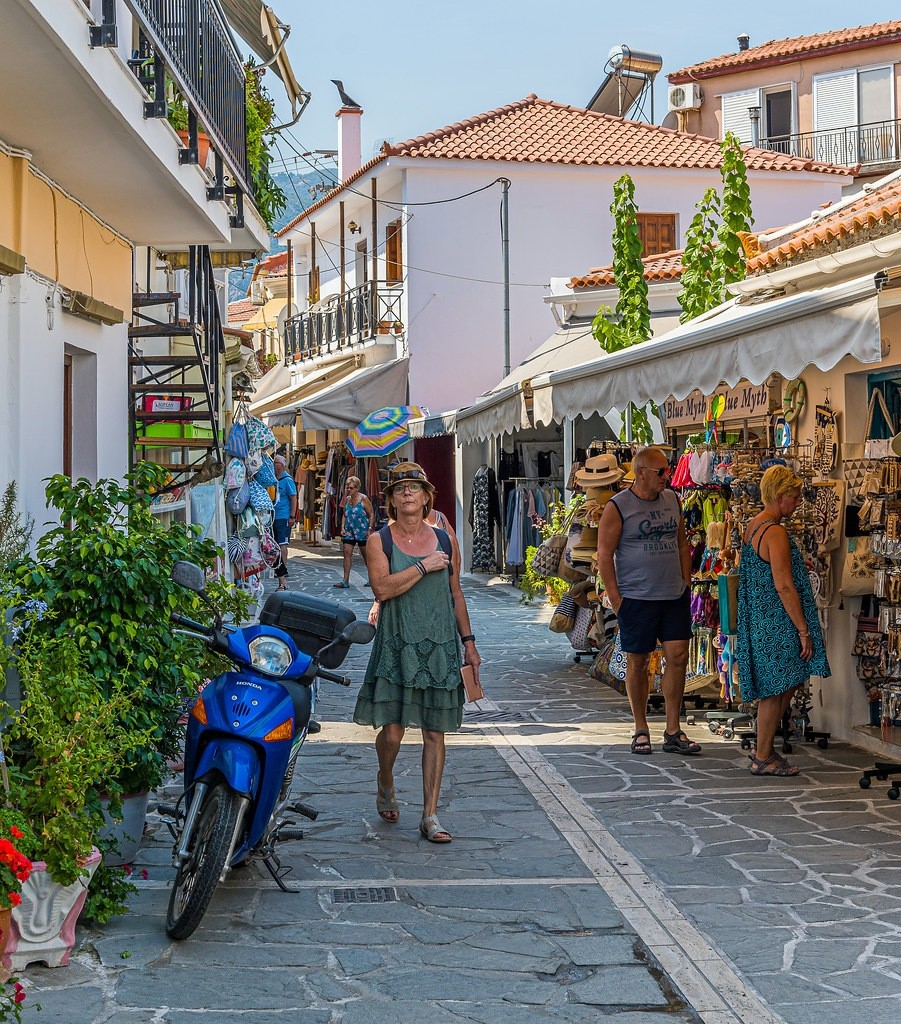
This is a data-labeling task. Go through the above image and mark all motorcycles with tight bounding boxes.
[157,559,379,943]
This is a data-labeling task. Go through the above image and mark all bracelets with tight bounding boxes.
[461,634,475,644]
[869,538,901,556]
[798,631,810,636]
[374,597,380,603]
[854,637,894,692]
[414,560,427,576]
[369,527,373,531]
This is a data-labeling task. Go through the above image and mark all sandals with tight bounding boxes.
[631,730,652,754]
[420,810,452,843]
[747,744,788,764]
[662,730,702,753]
[749,751,802,776]
[376,769,401,823]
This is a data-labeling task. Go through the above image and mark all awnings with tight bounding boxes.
[249,357,409,430]
[407,310,681,449]
[530,262,901,430]
[242,298,294,330]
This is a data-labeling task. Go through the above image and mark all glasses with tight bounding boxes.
[346,486,356,490]
[392,469,427,481]
[392,483,422,495]
[638,466,671,476]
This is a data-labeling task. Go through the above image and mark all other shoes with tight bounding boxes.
[274,573,289,577]
[364,582,371,587]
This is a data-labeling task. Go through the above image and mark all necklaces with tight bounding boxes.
[394,521,425,543]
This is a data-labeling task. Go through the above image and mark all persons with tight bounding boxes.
[264,456,297,592]
[736,464,832,777]
[353,462,481,842]
[597,448,702,754]
[333,476,375,588]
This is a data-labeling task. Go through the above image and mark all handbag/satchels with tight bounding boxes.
[659,633,719,693]
[841,387,901,680]
[530,501,582,577]
[549,580,627,696]
[717,572,739,636]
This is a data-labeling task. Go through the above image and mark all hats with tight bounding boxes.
[383,462,436,493]
[569,454,636,569]
[301,450,329,516]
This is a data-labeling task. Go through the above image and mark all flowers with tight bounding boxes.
[0,808,41,910]
[0,976,43,1024]
[0,588,163,924]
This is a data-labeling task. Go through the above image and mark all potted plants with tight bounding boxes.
[516,492,586,605]
[0,457,256,866]
[167,101,212,171]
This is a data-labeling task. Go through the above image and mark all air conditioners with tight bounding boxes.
[667,83,701,111]
[251,278,264,306]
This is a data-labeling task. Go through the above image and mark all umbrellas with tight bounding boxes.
[344,404,431,464]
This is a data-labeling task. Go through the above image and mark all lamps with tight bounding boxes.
[348,220,361,235]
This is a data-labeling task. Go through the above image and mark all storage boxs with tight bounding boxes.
[258,591,357,669]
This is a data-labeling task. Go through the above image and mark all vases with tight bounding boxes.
[2,843,103,971]
[0,906,11,985]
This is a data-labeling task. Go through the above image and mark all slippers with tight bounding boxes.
[275,583,288,592]
[333,581,350,588]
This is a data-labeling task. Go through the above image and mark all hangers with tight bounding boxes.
[542,445,551,452]
[528,444,540,452]
[504,441,513,448]
[515,478,564,493]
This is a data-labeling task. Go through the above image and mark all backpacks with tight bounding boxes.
[223,415,281,601]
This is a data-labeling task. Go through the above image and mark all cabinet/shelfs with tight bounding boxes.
[379,468,390,524]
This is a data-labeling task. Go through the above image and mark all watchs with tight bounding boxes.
[290,515,295,519]
